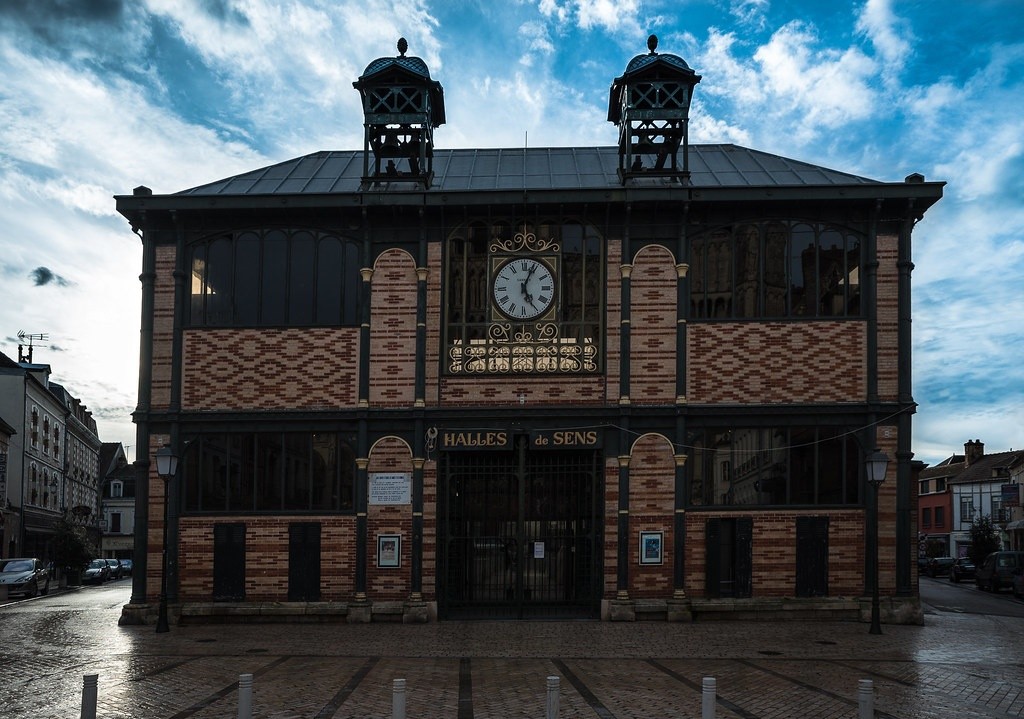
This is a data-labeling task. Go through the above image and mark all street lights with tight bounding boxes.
[865,449,893,633]
[153,443,180,632]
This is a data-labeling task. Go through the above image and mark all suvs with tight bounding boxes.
[0,558,49,599]
[106,559,124,580]
[119,559,134,577]
[82,561,106,585]
[94,559,112,581]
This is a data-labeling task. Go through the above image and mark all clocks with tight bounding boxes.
[492,259,558,321]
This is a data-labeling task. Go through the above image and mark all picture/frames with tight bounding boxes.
[638,529,665,567]
[376,533,401,569]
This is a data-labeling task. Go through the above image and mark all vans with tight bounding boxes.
[975,550,1024,593]
[926,557,957,578]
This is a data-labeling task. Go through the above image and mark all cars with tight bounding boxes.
[1011,566,1024,599]
[948,557,976,583]
